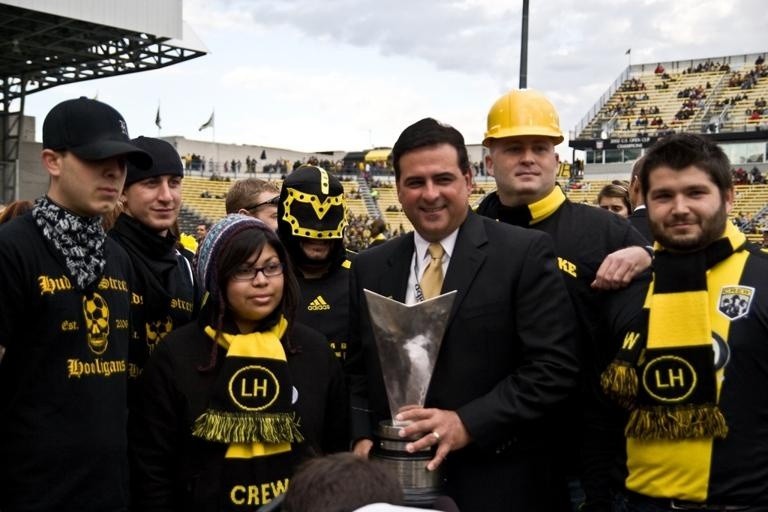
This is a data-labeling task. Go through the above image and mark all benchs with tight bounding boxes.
[578,64,768,138]
[557,177,768,258]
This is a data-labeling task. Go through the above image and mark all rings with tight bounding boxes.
[432,432,441,440]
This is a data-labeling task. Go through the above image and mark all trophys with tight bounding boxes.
[361,284,462,508]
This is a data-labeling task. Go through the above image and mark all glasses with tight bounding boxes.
[247,195,281,211]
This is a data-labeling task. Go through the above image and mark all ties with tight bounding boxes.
[420,242,445,300]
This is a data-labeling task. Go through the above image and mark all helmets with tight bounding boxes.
[482,90,565,148]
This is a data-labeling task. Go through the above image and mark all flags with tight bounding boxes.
[624,48,631,56]
[156,111,161,130]
[199,116,213,131]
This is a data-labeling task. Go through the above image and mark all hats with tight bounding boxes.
[194,212,279,292]
[42,96,153,172]
[125,136,184,189]
[278,165,348,241]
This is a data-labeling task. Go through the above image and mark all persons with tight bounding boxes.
[180,145,591,259]
[596,55,768,255]
[276,162,360,454]
[224,175,282,234]
[346,117,585,510]
[0,95,138,511]
[129,210,354,511]
[0,199,35,224]
[106,136,200,510]
[473,88,655,511]
[285,450,431,510]
[591,129,768,511]
